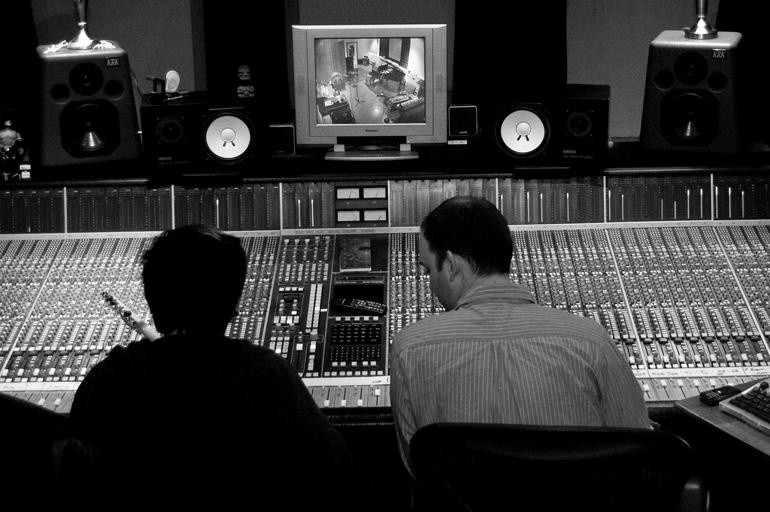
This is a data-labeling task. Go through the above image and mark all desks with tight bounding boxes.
[673,377,770,511]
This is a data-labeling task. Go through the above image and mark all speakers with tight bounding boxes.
[267,123,296,157]
[448,102,479,138]
[639,29,745,161]
[491,81,610,165]
[140,91,256,173]
[36,38,141,169]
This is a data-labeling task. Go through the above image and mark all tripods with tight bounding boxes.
[353,83,366,107]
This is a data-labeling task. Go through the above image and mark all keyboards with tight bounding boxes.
[718,377,770,434]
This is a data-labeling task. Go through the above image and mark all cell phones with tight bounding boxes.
[700,385,741,407]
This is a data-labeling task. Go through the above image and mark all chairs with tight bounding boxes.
[408,423,711,512]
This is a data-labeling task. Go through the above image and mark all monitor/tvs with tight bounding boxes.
[292,23,447,161]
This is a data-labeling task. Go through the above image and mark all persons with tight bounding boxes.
[385,194,659,483]
[70,222,352,512]
